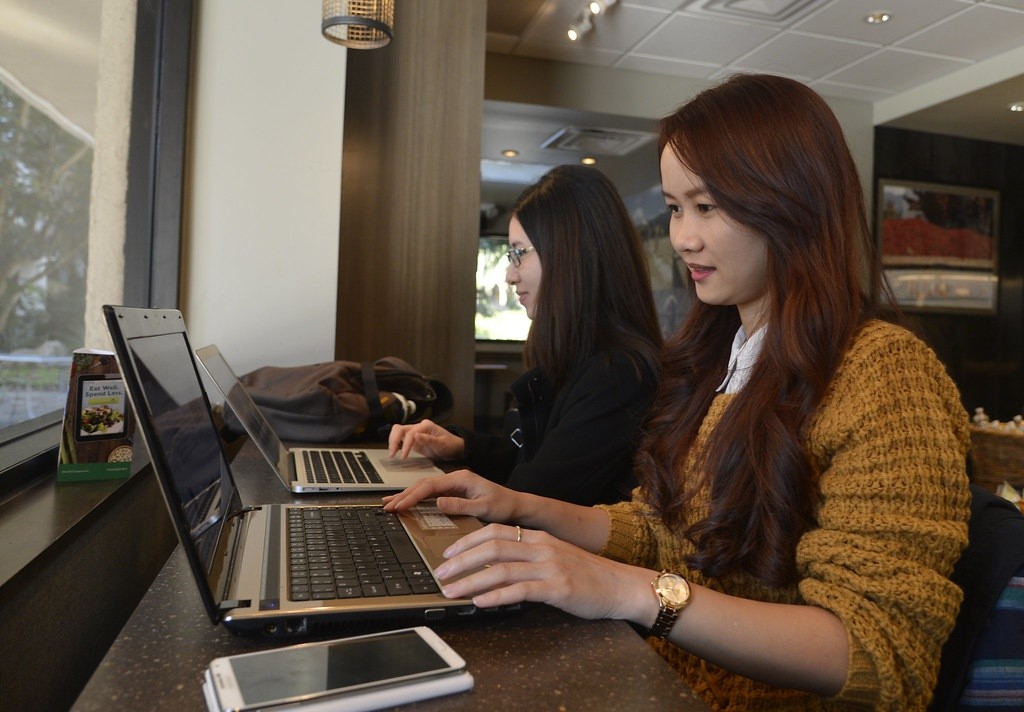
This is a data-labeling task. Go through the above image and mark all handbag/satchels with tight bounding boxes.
[221,357,455,446]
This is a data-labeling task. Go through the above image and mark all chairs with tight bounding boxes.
[927,482,1024,712]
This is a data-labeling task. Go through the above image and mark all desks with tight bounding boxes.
[67,439,713,712]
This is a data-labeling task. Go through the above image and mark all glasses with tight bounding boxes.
[507,245,536,268]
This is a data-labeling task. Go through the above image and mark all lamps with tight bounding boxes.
[590,0,617,16]
[567,7,596,41]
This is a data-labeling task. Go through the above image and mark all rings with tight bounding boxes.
[516,526,521,542]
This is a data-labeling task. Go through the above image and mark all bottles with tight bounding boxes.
[972,407,1024,433]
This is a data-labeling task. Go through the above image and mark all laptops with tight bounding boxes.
[194,344,448,493]
[102,304,523,639]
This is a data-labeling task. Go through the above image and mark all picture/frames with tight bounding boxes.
[875,177,1002,270]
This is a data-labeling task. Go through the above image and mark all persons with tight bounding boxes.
[380,72,972,712]
[386,165,664,506]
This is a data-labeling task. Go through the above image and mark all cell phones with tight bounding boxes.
[210,626,467,712]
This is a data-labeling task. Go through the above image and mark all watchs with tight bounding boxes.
[648,570,691,640]
